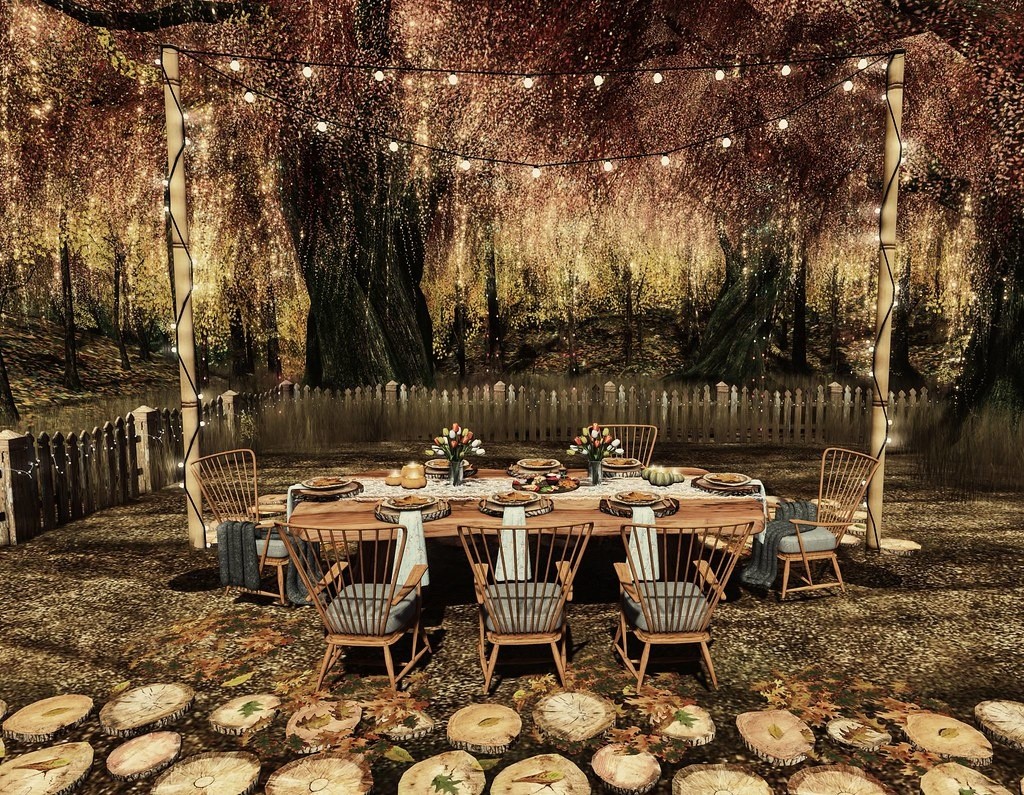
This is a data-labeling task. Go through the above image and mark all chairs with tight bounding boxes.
[457,521,594,694]
[273,520,430,699]
[612,521,755,694]
[741,448,881,599]
[587,425,658,468]
[188,449,331,605]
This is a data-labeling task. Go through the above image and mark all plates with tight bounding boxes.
[602,458,641,467]
[425,458,470,469]
[302,477,353,488]
[610,490,664,504]
[518,458,560,468]
[487,491,541,505]
[703,473,752,486]
[382,494,435,509]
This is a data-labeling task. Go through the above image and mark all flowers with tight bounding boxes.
[565,423,624,462]
[424,423,485,466]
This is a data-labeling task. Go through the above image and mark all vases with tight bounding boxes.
[449,462,464,486]
[588,461,602,485]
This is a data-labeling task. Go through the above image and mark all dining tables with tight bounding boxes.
[289,466,764,590]
